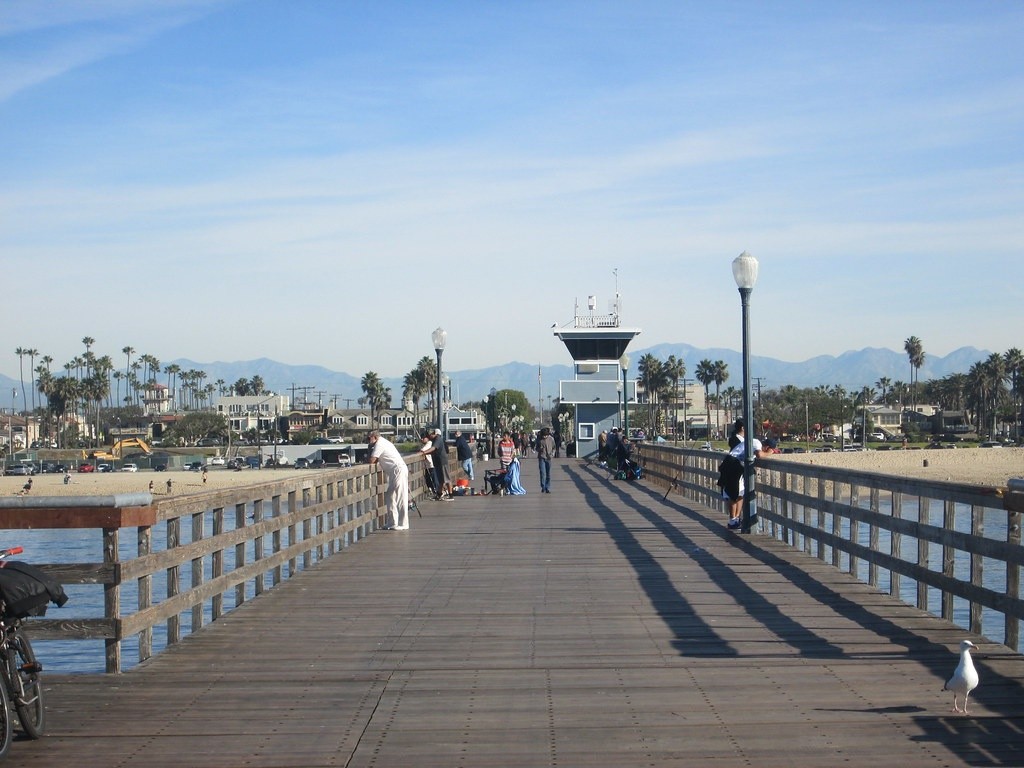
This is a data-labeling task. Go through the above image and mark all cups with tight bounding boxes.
[500,489,503,497]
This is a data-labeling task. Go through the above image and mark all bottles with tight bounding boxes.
[471,488,474,495]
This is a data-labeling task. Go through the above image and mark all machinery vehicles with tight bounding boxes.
[87,436,154,465]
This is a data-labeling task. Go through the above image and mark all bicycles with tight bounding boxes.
[0,544,46,768]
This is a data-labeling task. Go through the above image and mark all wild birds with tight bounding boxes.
[940,639,979,716]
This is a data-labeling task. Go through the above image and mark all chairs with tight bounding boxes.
[485,458,518,495]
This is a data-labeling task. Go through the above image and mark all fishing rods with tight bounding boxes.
[585,454,598,468]
[606,471,613,480]
[662,422,706,501]
[414,430,439,499]
[408,487,424,519]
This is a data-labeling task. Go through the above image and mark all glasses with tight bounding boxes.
[368,436,372,440]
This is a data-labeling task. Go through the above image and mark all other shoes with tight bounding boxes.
[542,487,546,493]
[467,476,474,480]
[728,520,743,529]
[393,526,409,530]
[444,498,455,502]
[440,491,446,498]
[381,524,394,530]
[546,488,550,493]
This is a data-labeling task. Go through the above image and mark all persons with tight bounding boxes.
[598,427,636,471]
[498,431,515,469]
[536,428,556,493]
[729,418,745,452]
[23,478,32,493]
[64,474,72,484]
[511,430,560,458]
[201,473,207,482]
[719,438,780,529]
[420,432,436,496]
[149,480,154,492]
[367,430,409,531]
[417,430,455,502]
[451,430,474,480]
[167,479,172,492]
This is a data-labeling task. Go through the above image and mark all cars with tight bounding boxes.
[309,435,344,445]
[182,461,208,472]
[5,462,70,476]
[196,433,288,447]
[77,463,94,473]
[121,463,138,473]
[337,453,351,467]
[777,431,886,454]
[154,463,167,471]
[228,453,326,470]
[922,430,1018,449]
[97,463,112,472]
[211,456,225,466]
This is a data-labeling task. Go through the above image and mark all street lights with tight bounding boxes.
[483,395,489,454]
[511,403,525,457]
[731,247,760,534]
[615,380,624,430]
[117,416,122,467]
[431,325,447,451]
[618,353,631,442]
[441,372,450,442]
[490,387,497,459]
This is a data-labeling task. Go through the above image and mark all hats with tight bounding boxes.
[435,428,441,435]
